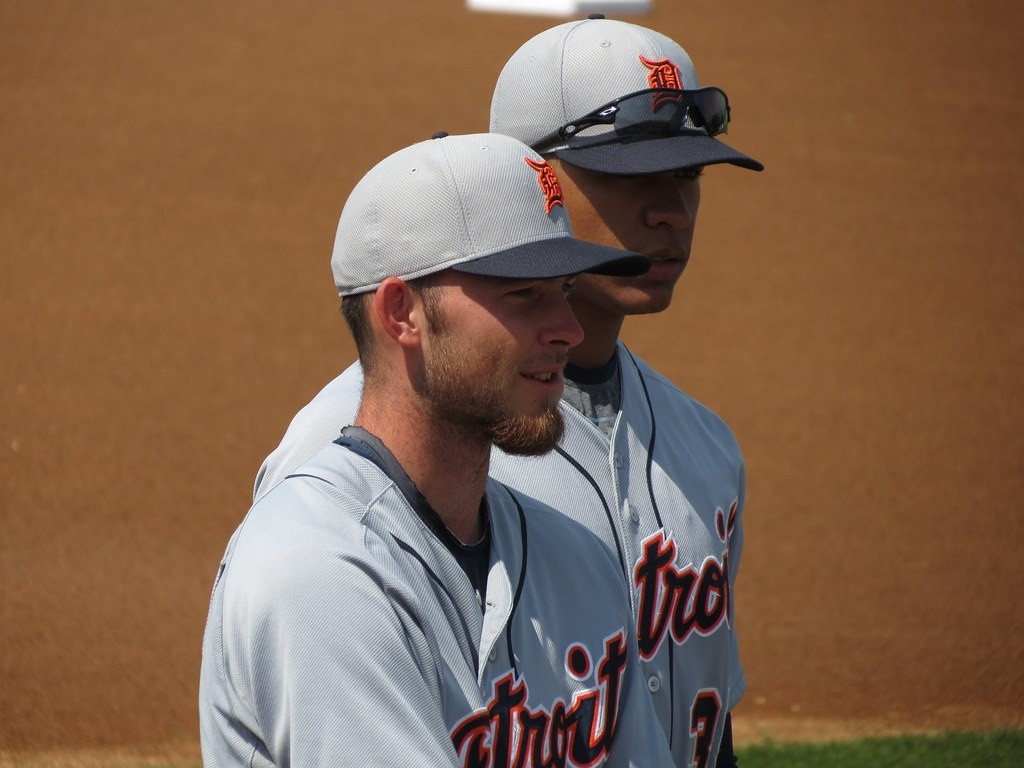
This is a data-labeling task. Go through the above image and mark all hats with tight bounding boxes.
[328,132,650,298]
[487,14,765,174]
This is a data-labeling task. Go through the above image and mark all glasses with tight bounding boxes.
[535,87,734,151]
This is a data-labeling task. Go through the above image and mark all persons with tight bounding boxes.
[197,133,676,768]
[247,15,764,768]
